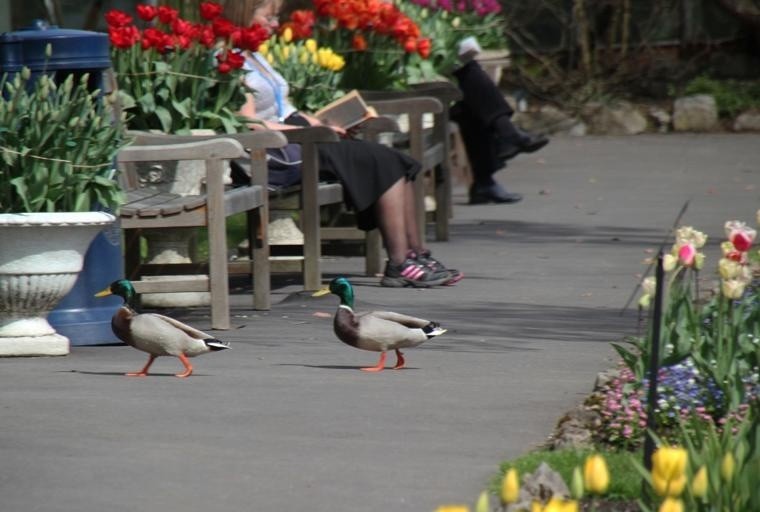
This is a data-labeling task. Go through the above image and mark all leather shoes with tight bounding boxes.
[499,132,548,159]
[470,184,520,203]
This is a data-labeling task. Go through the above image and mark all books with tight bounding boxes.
[459,37,482,64]
[314,89,378,136]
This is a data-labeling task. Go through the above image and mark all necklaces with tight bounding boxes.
[250,61,287,120]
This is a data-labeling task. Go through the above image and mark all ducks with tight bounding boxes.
[310,275,448,373]
[95,278,232,379]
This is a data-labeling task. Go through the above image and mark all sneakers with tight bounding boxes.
[381,256,452,287]
[407,248,463,284]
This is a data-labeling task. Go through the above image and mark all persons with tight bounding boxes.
[219,0,466,290]
[435,52,551,205]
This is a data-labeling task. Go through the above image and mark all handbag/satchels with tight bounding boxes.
[267,143,303,188]
